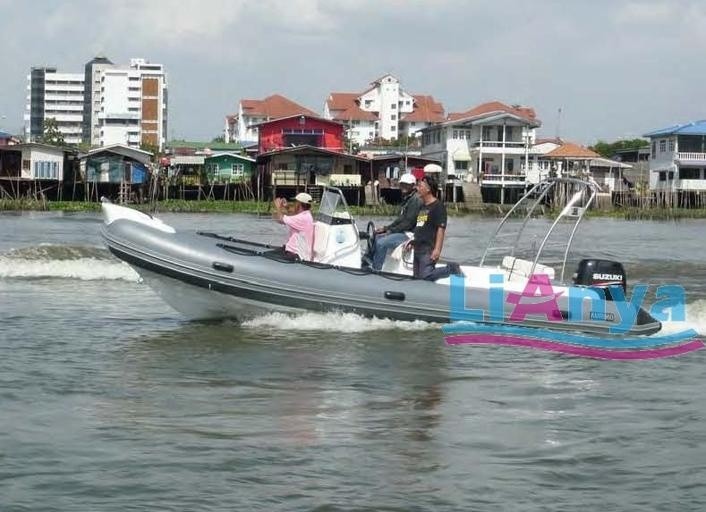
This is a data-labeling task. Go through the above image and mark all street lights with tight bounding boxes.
[0,116,7,119]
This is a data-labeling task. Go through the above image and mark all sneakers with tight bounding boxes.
[447,261,461,274]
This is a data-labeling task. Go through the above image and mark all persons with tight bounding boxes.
[361,172,422,276]
[401,175,462,282]
[269,192,315,261]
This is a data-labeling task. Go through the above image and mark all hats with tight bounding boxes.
[398,172,417,185]
[290,192,313,205]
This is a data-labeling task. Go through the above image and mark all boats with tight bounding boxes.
[92,176,669,339]
